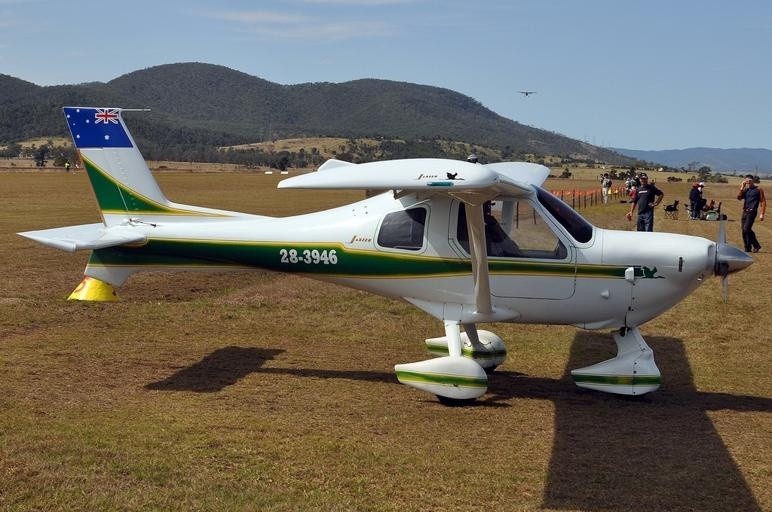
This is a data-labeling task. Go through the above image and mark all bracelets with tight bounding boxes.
[760,212,765,214]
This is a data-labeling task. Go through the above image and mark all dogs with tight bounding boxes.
[664,200,679,211]
[703,200,716,211]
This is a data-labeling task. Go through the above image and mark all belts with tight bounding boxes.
[744,209,754,211]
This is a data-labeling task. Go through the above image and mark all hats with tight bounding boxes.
[639,173,648,179]
[633,177,639,181]
[692,183,700,187]
[604,173,609,175]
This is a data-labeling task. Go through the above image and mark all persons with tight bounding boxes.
[751,175,761,188]
[736,174,767,254]
[688,181,727,221]
[624,173,664,231]
[598,165,656,206]
[64,159,83,176]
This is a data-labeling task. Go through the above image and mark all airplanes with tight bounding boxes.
[17,105,754,408]
[519,90,536,97]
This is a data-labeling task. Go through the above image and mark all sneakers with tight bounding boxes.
[745,245,761,253]
[690,217,696,220]
